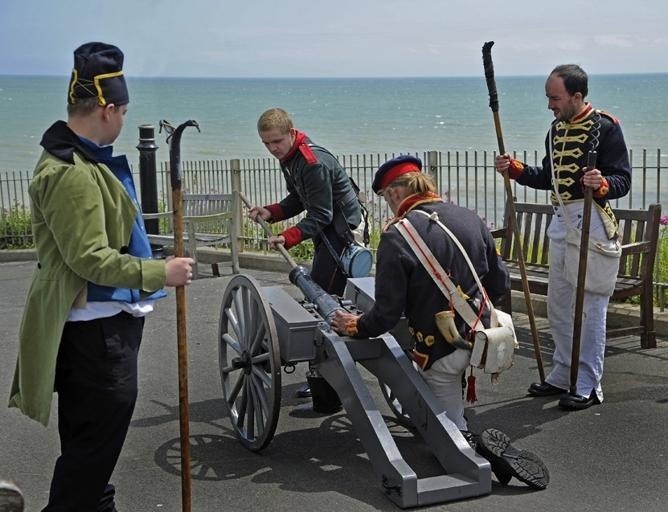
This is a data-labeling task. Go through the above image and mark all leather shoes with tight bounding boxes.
[476,429,550,491]
[558,391,596,412]
[295,384,312,398]
[526,381,568,396]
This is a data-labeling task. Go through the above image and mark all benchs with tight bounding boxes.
[489,195,662,355]
[141,188,241,281]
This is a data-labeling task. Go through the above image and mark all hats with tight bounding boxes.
[67,42,130,106]
[371,155,423,197]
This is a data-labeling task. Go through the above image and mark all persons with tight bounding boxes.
[495,64,632,410]
[248,107,362,397]
[6,41,195,512]
[329,155,550,492]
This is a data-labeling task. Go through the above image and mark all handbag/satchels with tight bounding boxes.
[469,326,514,375]
[350,208,372,251]
[490,308,519,351]
[562,227,623,298]
